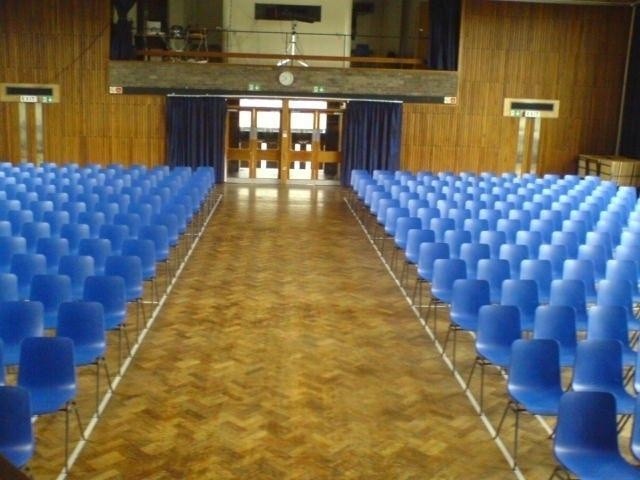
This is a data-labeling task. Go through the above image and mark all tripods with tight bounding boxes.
[278,33,309,67]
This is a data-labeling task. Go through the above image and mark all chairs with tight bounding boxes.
[182,23,209,64]
